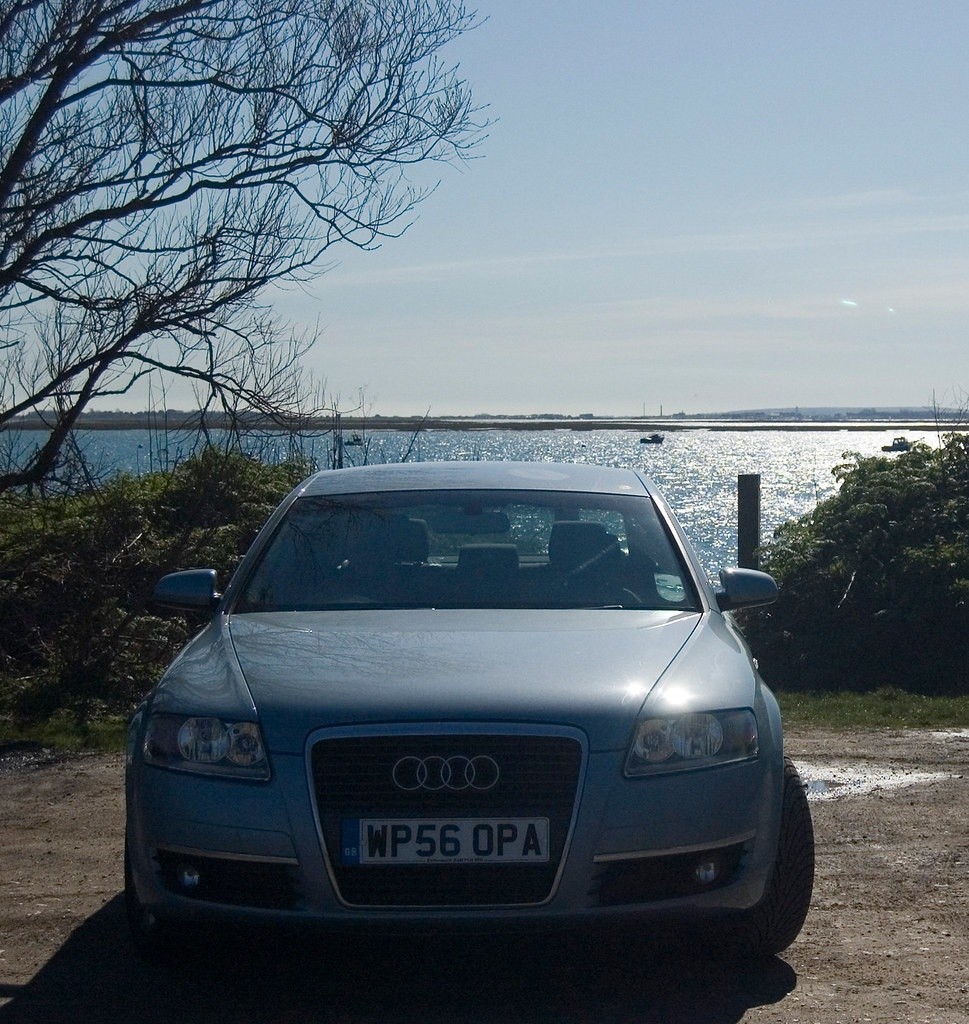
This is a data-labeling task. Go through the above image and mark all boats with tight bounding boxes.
[881,437,912,452]
[639,434,665,444]
[344,434,364,446]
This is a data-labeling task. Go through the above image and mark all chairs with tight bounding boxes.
[306,513,659,614]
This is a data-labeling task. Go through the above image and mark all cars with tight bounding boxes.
[122,460,816,972]
